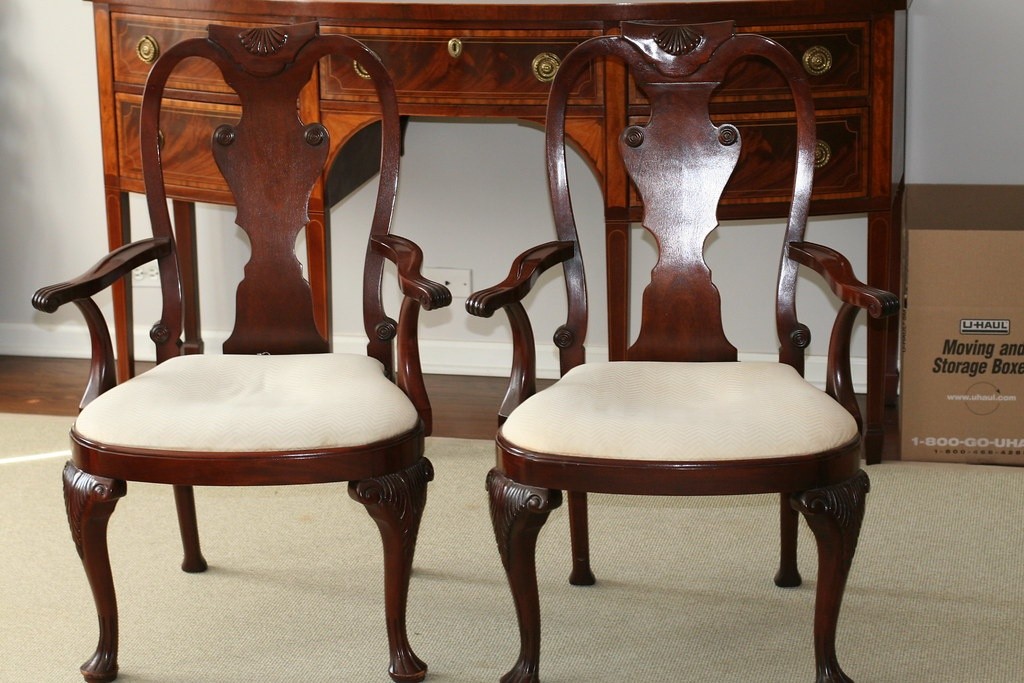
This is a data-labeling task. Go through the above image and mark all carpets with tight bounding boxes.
[0,409,1024,679]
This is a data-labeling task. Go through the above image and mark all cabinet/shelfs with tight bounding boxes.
[84,0,915,465]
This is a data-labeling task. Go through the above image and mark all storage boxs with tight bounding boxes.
[896,182,1024,467]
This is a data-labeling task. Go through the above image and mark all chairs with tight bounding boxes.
[30,22,450,681]
[464,24,896,683]
